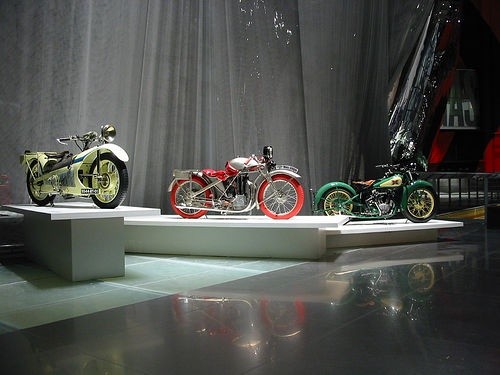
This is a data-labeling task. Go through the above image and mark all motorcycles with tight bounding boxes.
[330,258,455,323]
[18,117,130,208]
[169,282,308,352]
[309,163,440,225]
[167,142,305,220]
